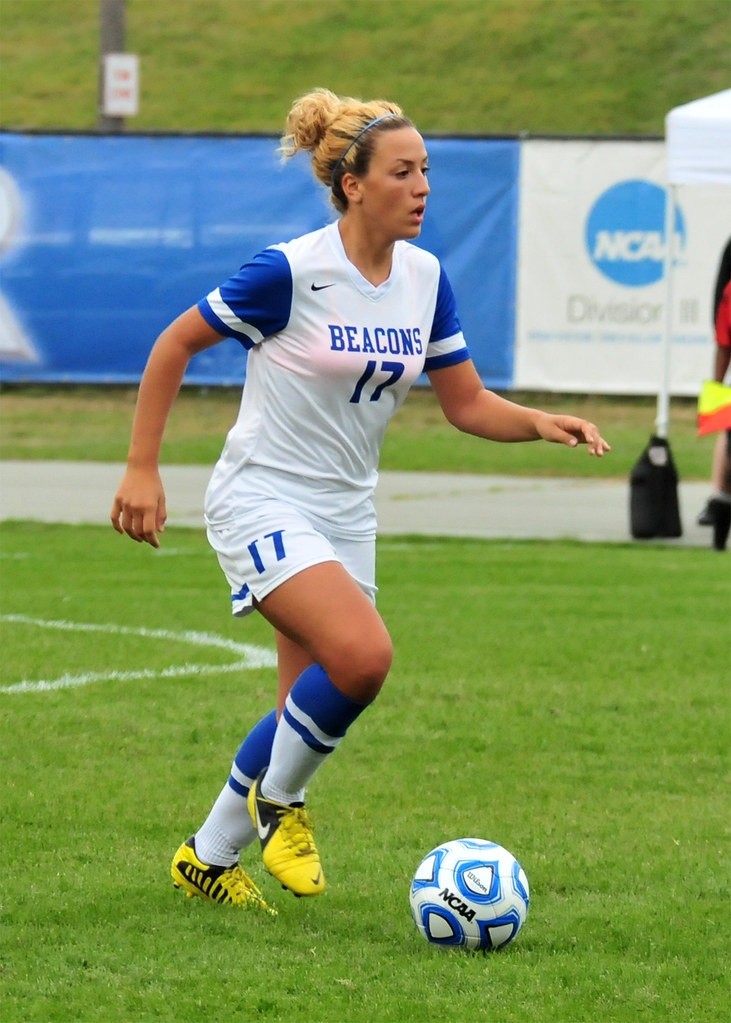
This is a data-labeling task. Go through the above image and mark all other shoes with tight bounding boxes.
[696,496,731,551]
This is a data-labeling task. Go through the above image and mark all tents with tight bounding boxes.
[654,88,731,437]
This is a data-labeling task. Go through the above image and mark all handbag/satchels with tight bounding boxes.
[630,436,683,539]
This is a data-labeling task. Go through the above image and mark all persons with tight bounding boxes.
[113,87,612,916]
[698,240,731,525]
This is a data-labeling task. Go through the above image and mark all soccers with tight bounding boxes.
[407,836,532,951]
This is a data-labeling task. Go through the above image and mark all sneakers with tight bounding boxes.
[169,833,278,921]
[245,766,326,898]
